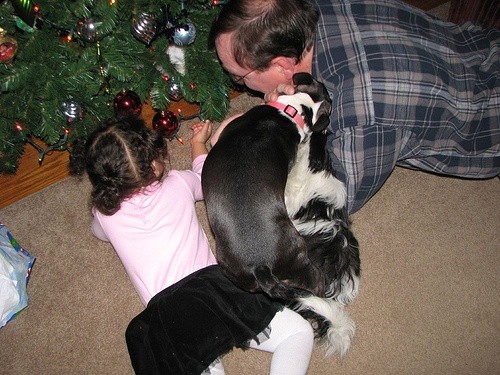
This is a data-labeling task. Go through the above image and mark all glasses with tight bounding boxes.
[229,60,267,86]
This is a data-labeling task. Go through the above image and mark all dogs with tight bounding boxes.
[200,72,361,359]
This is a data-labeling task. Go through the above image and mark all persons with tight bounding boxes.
[207,0,500,216]
[67,113,315,375]
